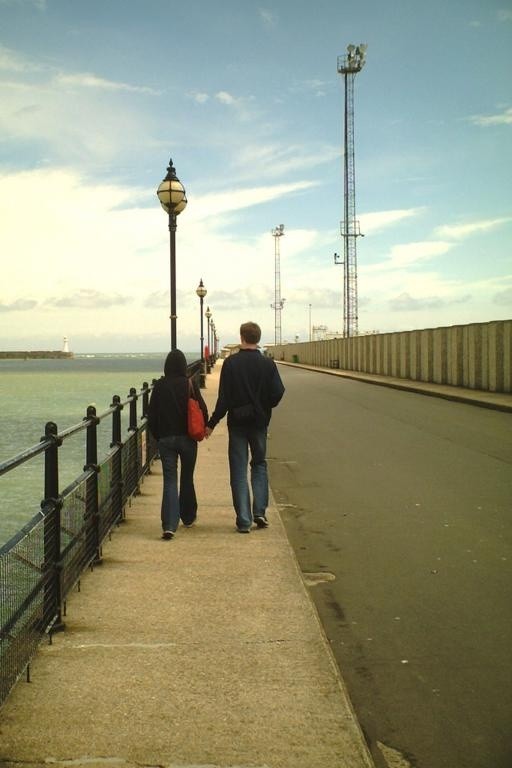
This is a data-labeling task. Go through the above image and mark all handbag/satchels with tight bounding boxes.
[187,398,205,441]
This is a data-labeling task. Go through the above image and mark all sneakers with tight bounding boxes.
[184,515,197,527]
[163,530,175,539]
[236,526,251,533]
[253,516,269,528]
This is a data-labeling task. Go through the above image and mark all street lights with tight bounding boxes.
[158,158,187,351]
[195,279,207,360]
[205,307,218,355]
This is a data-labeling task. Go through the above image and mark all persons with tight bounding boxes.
[205,322,285,532]
[149,350,210,539]
